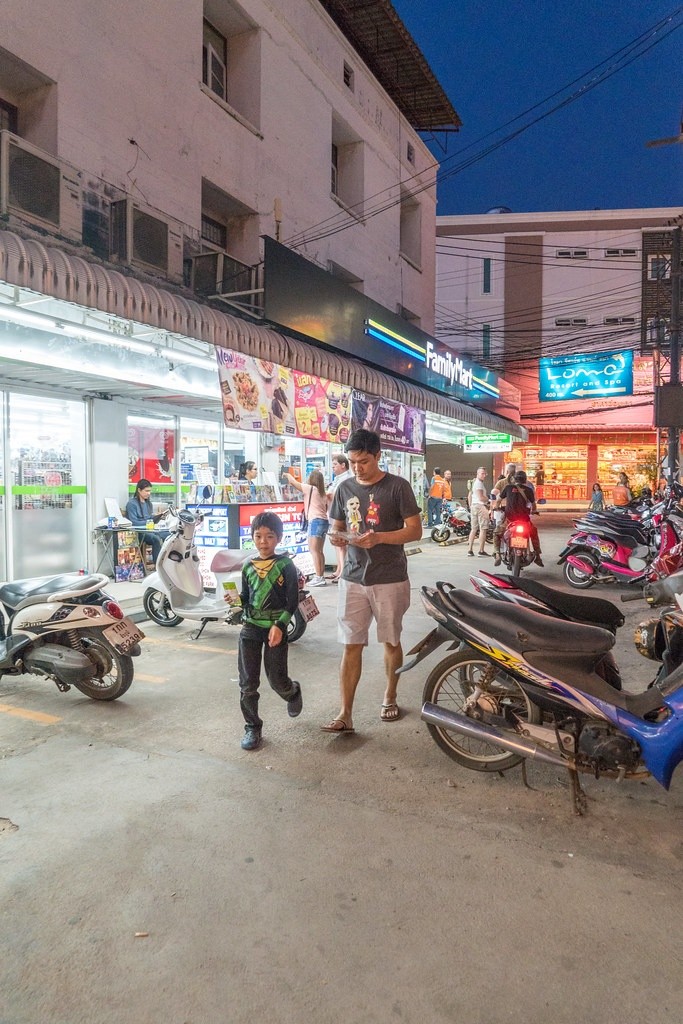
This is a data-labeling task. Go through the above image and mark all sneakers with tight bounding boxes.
[305,575,326,587]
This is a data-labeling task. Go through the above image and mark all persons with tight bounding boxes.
[612,472,634,506]
[641,484,651,497]
[321,428,422,733]
[324,453,353,583]
[466,467,491,557]
[223,512,303,749]
[423,467,453,529]
[492,463,544,568]
[588,483,607,511]
[282,470,329,586]
[535,466,546,484]
[126,479,171,561]
[238,461,258,485]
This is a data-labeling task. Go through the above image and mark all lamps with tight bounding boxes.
[0,303,219,369]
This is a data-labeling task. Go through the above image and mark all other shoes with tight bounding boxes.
[494,557,501,566]
[467,550,474,556]
[534,557,544,568]
[478,551,491,557]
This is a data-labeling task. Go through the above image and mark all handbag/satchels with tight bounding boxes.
[299,509,308,532]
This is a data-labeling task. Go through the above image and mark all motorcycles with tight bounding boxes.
[395,568,683,817]
[430,506,497,544]
[555,482,683,589]
[486,494,546,578]
[142,484,322,644]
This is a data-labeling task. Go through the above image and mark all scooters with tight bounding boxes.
[0,570,145,701]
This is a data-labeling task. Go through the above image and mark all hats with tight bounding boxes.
[516,471,527,478]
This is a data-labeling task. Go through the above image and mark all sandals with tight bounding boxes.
[287,682,302,718]
[332,576,340,582]
[241,727,263,750]
[324,575,336,579]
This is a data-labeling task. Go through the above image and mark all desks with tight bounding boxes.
[94,526,176,582]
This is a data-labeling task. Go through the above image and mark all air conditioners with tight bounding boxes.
[190,250,256,315]
[0,130,87,246]
[107,196,180,282]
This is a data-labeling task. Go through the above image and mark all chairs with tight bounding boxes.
[112,511,152,581]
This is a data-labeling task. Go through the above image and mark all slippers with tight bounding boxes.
[319,719,355,733]
[379,700,399,722]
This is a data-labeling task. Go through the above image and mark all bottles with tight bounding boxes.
[150,520,154,529]
[146,520,150,530]
[161,518,166,529]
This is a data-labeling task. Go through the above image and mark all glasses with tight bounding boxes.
[249,467,257,472]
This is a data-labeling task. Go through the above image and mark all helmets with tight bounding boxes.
[634,619,664,662]
